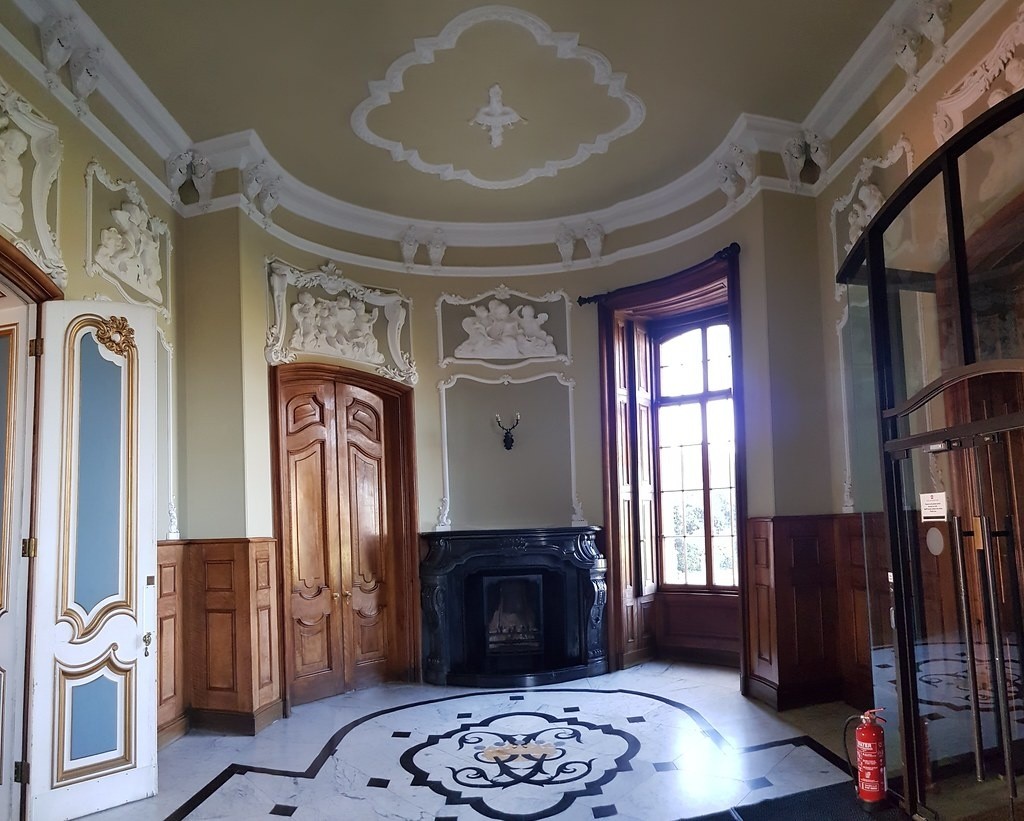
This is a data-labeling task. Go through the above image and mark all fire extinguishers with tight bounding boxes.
[840,705,893,802]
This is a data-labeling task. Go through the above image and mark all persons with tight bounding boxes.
[112,202,164,283]
[470,299,549,342]
[844,184,887,253]
[0,117,28,215]
[988,61,1023,109]
[292,291,378,364]
